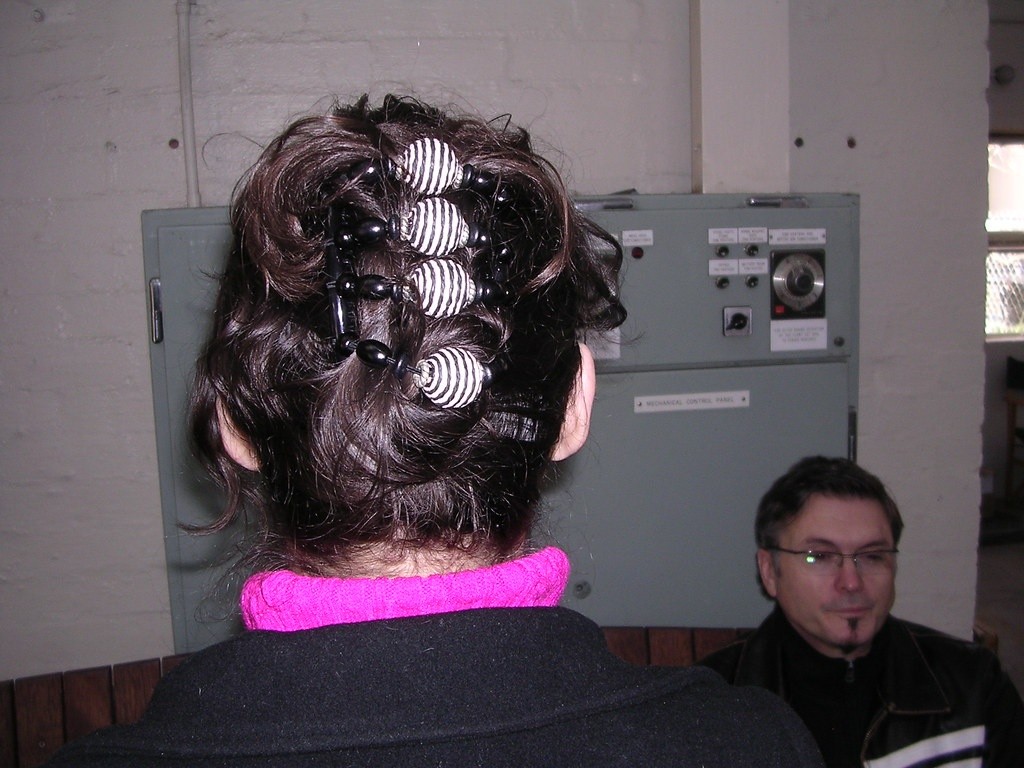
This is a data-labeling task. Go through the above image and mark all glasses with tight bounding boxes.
[771,548,899,577]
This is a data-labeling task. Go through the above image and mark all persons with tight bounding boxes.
[701,453,1024,768]
[0,92,823,767]
